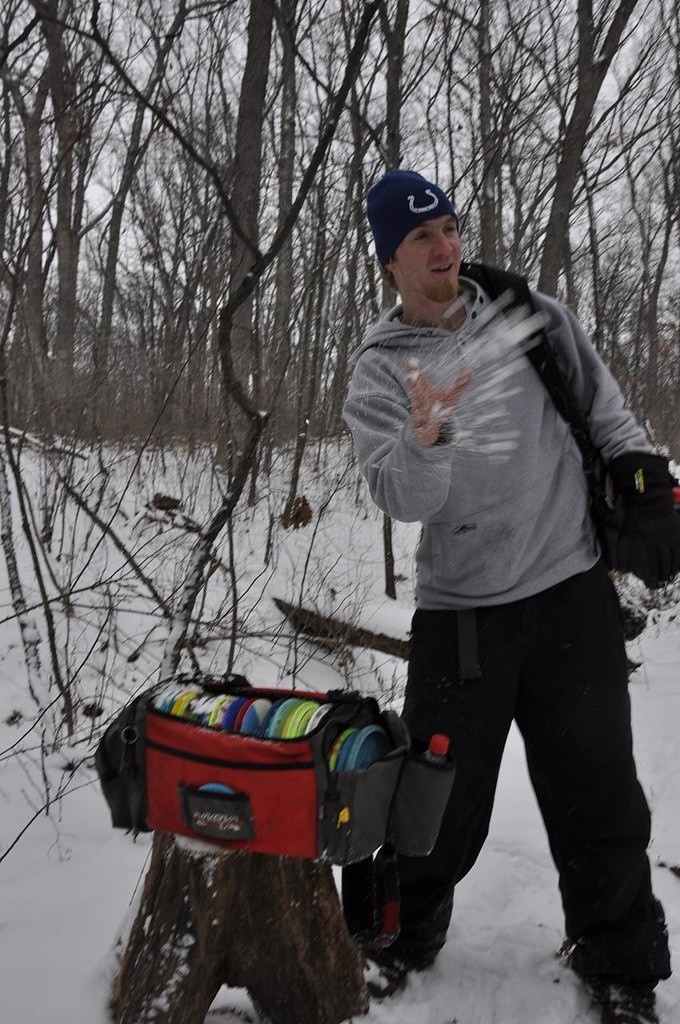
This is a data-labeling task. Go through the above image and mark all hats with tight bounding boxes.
[366,170,461,264]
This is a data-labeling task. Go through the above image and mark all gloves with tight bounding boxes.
[607,450,678,588]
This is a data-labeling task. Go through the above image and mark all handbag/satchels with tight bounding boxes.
[95,673,455,866]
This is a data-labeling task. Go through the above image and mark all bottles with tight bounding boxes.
[420,733,450,765]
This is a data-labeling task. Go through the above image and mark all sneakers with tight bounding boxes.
[596,985,656,1024]
[364,952,436,996]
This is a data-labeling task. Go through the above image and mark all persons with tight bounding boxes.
[338,164,674,1024]
